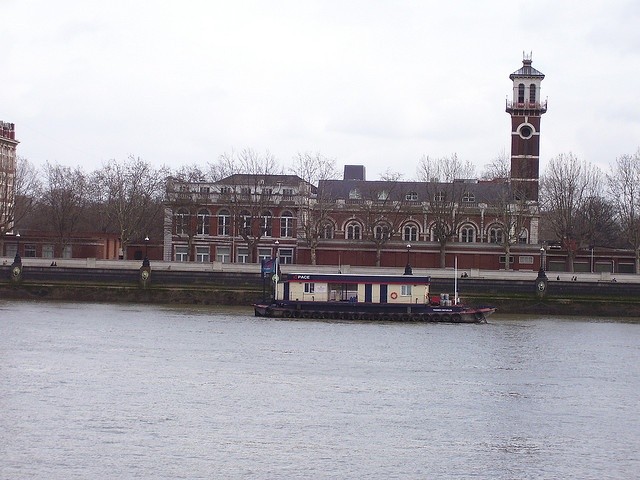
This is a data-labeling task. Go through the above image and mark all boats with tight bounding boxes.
[249,273,500,324]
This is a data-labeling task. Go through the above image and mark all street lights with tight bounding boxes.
[14,233,21,263]
[142,237,150,267]
[536,248,548,279]
[273,239,281,273]
[404,244,413,275]
